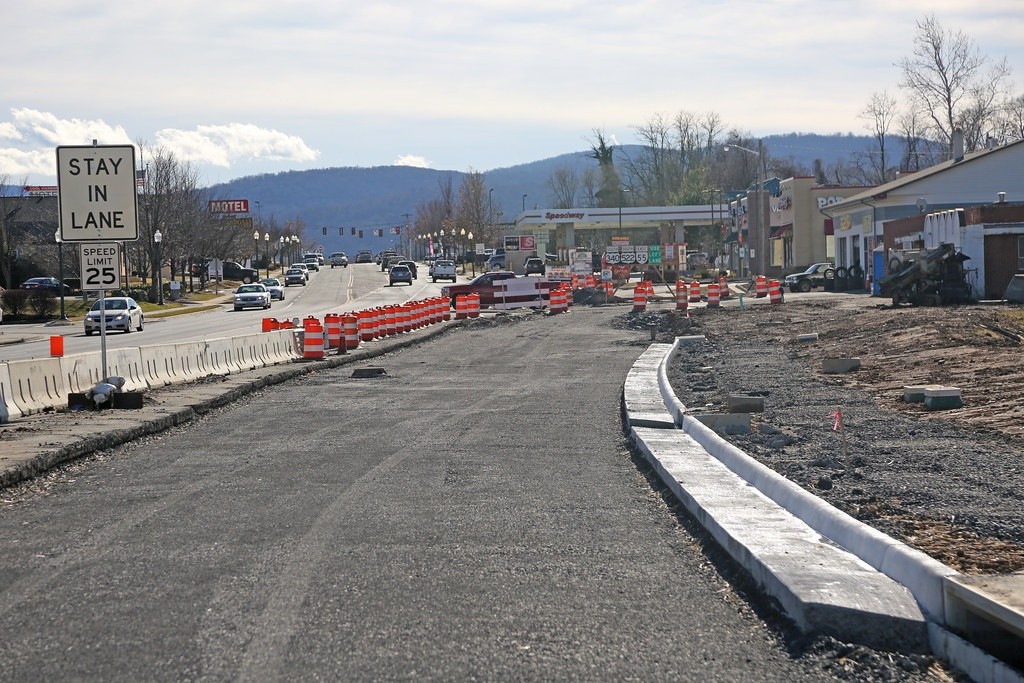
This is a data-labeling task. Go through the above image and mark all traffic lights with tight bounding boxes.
[396,227,400,235]
[379,230,383,237]
[359,230,363,238]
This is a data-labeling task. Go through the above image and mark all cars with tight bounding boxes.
[686,250,709,270]
[428,262,434,276]
[290,264,309,281]
[284,268,307,286]
[374,249,419,286]
[259,278,285,300]
[232,284,271,310]
[303,253,324,265]
[84,297,144,336]
[457,249,506,270]
[535,250,557,262]
[354,249,372,263]
[20,276,70,296]
[304,258,320,272]
[328,251,348,268]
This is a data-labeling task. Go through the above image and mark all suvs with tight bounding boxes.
[523,257,546,276]
[784,263,833,293]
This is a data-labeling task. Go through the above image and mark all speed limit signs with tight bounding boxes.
[79,242,121,290]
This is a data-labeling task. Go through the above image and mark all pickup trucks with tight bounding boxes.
[441,269,550,310]
[432,260,457,283]
[424,252,457,262]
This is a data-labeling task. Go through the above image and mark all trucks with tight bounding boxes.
[191,261,259,284]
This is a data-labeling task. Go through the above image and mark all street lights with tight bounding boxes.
[265,233,269,278]
[468,232,475,278]
[618,190,630,248]
[489,188,494,246]
[523,194,527,212]
[701,145,766,275]
[415,228,465,272]
[280,235,300,274]
[254,231,259,283]
